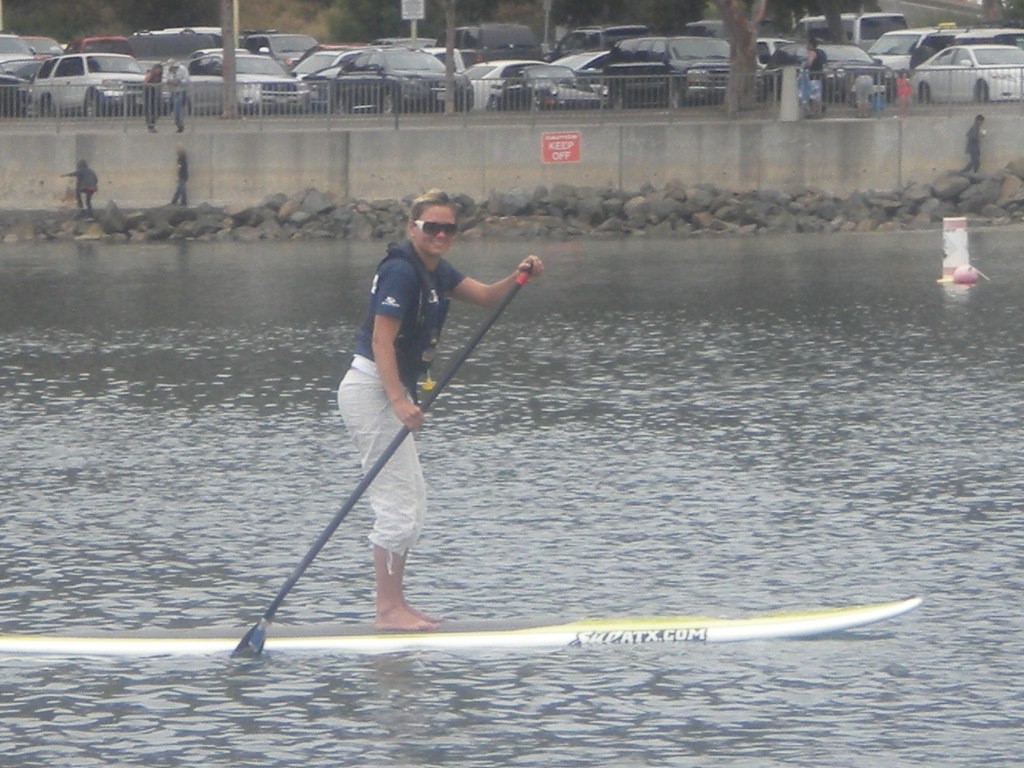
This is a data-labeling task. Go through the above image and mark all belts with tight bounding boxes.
[351,357,413,404]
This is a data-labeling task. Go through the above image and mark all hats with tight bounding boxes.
[167,59,179,70]
[774,41,787,49]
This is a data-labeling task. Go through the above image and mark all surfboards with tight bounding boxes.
[0,588,924,662]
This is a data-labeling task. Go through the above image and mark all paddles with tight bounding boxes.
[228,263,538,664]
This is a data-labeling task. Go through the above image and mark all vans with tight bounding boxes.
[789,12,909,52]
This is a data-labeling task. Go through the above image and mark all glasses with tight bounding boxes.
[414,220,458,236]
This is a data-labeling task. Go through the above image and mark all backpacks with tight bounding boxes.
[811,49,826,64]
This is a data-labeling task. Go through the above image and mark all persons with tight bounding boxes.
[145,63,163,132]
[896,68,910,115]
[171,145,187,205]
[962,114,985,172]
[166,59,189,132]
[803,39,822,111]
[60,159,97,208]
[337,189,543,630]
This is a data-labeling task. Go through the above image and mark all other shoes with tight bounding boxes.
[148,127,157,133]
[177,126,184,133]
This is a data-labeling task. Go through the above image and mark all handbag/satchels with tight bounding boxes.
[84,184,96,192]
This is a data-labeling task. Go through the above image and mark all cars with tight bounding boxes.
[336,48,475,114]
[289,50,362,80]
[461,60,549,111]
[551,49,611,86]
[606,37,774,110]
[867,24,938,75]
[756,38,790,62]
[30,54,147,119]
[20,35,64,60]
[0,60,45,116]
[302,59,348,113]
[138,60,188,114]
[762,42,897,104]
[246,33,318,69]
[294,44,345,68]
[520,64,608,110]
[183,54,310,115]
[369,39,437,49]
[189,48,250,60]
[910,45,1024,102]
[63,35,135,56]
[0,34,37,63]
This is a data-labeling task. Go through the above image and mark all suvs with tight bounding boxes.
[434,23,542,67]
[543,25,651,62]
[909,20,1024,72]
